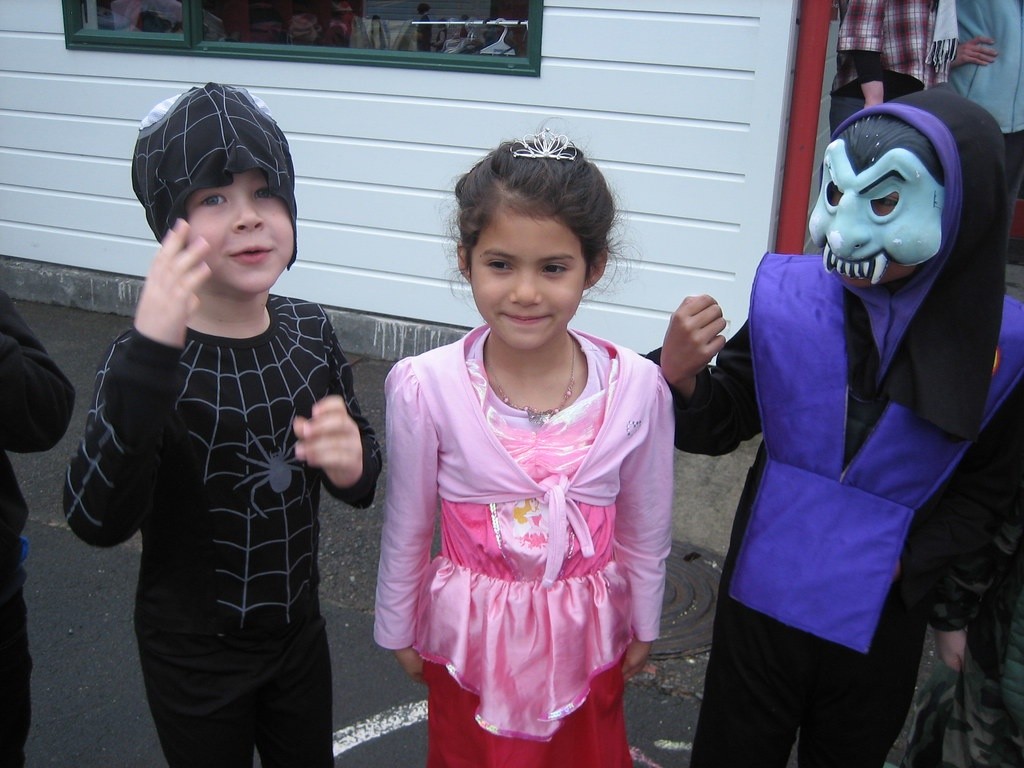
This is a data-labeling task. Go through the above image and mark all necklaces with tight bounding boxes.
[486,338,576,426]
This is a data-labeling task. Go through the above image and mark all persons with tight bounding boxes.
[374,141,674,768]
[62,83,382,768]
[0,288,75,768]
[829,0,1023,290]
[638,86,1024,768]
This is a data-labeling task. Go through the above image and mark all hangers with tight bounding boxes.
[441,18,528,57]
